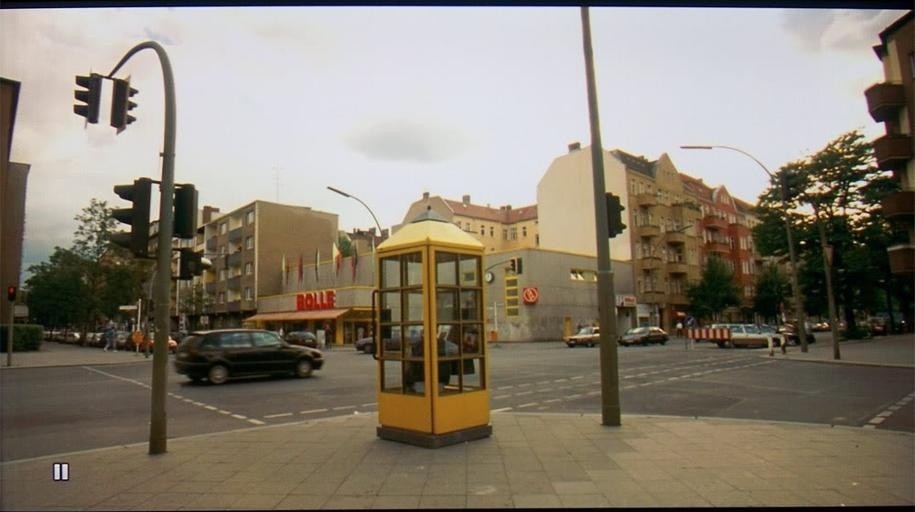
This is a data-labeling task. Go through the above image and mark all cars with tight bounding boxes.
[43,326,188,355]
[767,310,906,345]
[563,326,600,347]
[355,329,424,354]
[285,330,318,347]
[174,327,324,386]
[617,326,669,347]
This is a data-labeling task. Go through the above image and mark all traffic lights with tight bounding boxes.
[606,192,628,238]
[109,176,149,259]
[509,258,517,273]
[73,72,102,124]
[8,285,17,301]
[179,247,212,282]
[111,77,140,134]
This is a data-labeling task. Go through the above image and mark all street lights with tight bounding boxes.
[679,144,808,352]
[327,185,388,332]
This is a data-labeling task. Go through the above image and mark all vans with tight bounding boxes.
[710,322,786,348]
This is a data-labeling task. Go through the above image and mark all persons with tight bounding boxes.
[102,319,118,352]
[675,320,683,337]
[326,323,334,352]
[409,327,459,391]
